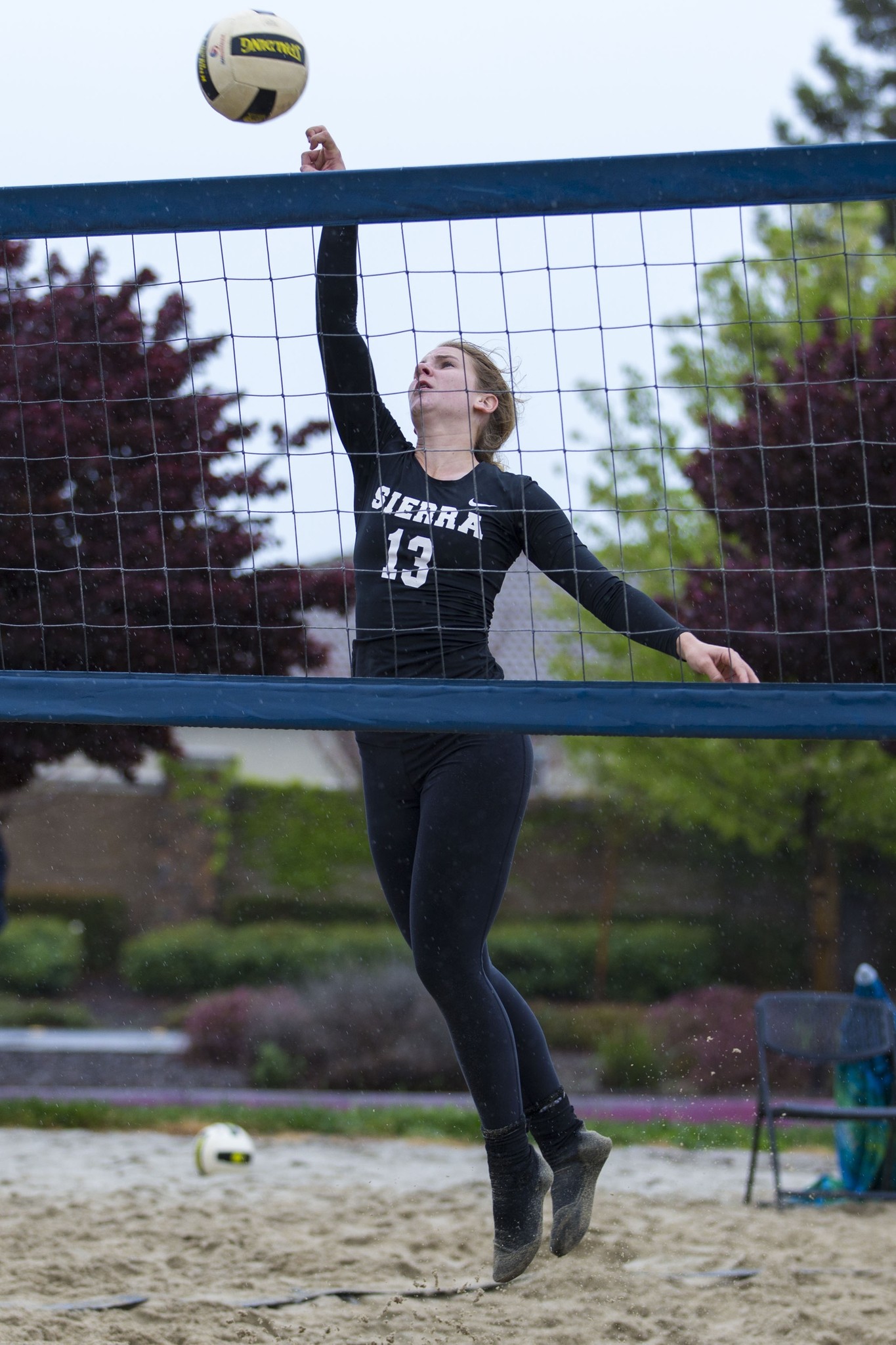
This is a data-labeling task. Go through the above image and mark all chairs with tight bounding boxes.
[743,989,896,1213]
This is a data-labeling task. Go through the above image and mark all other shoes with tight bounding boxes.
[547,1124,614,1259]
[480,1153,553,1289]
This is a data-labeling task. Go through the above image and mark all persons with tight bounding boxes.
[298,126,762,1285]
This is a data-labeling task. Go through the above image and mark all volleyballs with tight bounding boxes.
[190,1120,257,1176]
[195,0,310,125]
